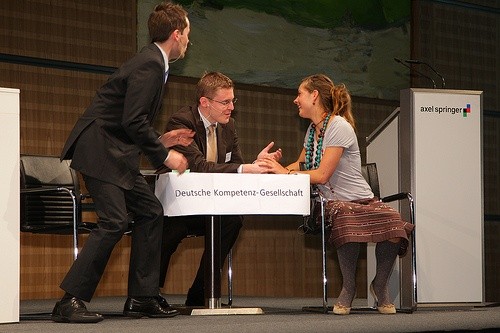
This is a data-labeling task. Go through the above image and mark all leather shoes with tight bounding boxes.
[187,288,227,309]
[50,296,104,324]
[124,296,184,318]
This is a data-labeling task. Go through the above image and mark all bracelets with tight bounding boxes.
[286,170,292,175]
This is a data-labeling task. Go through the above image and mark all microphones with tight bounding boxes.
[394,58,436,89]
[209,104,222,113]
[404,60,446,89]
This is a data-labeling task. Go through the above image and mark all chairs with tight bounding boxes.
[299,162,417,314]
[20,154,233,320]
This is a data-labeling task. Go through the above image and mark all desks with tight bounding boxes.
[154,172,310,316]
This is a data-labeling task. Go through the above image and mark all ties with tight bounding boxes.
[207,125,218,163]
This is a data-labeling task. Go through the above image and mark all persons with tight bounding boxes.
[260,75,413,314]
[51,1,189,324]
[156,72,282,307]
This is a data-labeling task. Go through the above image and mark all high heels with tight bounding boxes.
[370,280,396,314]
[333,287,357,315]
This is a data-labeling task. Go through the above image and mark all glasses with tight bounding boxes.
[207,97,238,104]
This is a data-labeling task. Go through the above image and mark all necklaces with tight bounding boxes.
[305,111,331,171]
